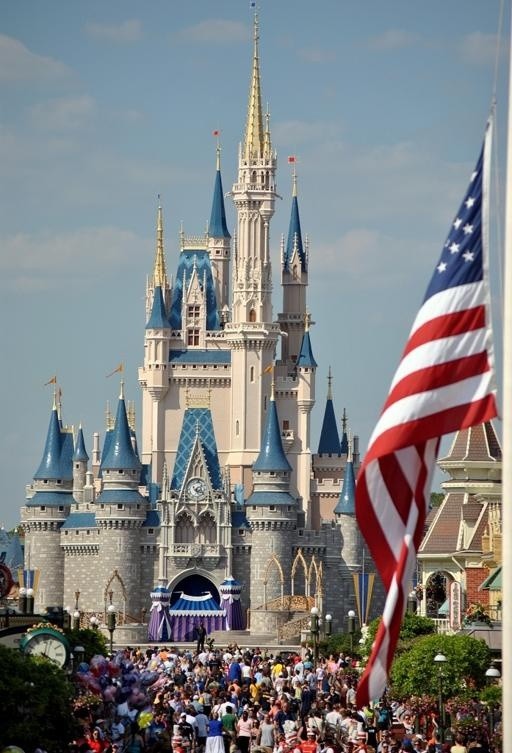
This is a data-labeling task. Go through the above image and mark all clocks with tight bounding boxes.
[18,620,72,670]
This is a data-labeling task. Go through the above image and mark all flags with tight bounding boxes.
[348,110,499,709]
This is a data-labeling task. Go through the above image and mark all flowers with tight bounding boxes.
[461,601,496,629]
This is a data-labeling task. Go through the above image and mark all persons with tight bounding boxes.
[55,631,491,753]
[195,621,207,651]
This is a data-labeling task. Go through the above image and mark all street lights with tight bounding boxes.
[346,609,359,652]
[74,588,80,612]
[309,607,320,660]
[432,649,449,741]
[73,610,99,631]
[323,614,334,641]
[107,605,116,654]
[19,586,35,615]
[485,661,501,734]
[109,588,114,604]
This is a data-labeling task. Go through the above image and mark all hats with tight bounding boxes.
[356,732,366,739]
[193,695,199,699]
[382,730,390,736]
[181,712,186,717]
[306,728,316,736]
[174,691,180,697]
[112,743,119,749]
[288,731,298,741]
[96,719,106,723]
[172,735,183,743]
